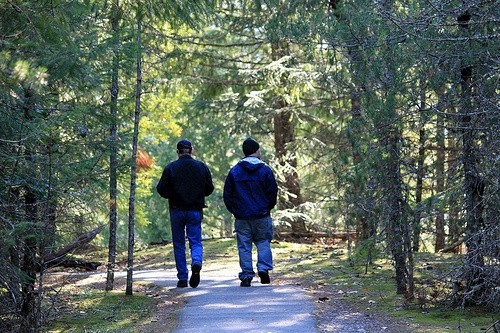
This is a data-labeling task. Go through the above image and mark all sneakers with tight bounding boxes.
[189,264,200,288]
[240,278,251,287]
[177,280,187,288]
[258,270,270,284]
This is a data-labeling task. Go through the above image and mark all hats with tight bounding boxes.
[177,140,191,149]
[242,138,260,156]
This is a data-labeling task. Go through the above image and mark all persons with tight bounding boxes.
[222,137,279,288]
[156,139,215,288]
